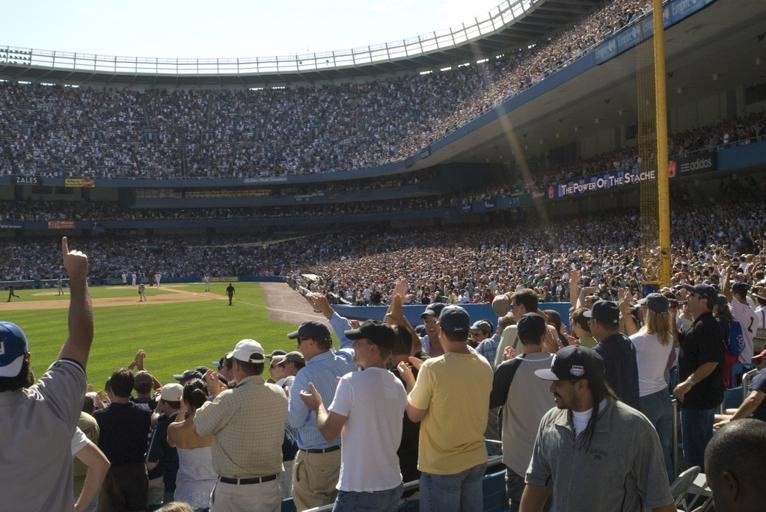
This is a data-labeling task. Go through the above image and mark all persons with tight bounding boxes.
[5,78,373,277]
[155,271,161,287]
[265,321,358,511]
[565,265,766,512]
[1,234,96,511]
[519,343,677,511]
[57,277,65,296]
[67,347,218,511]
[7,284,20,302]
[202,272,210,292]
[148,270,154,286]
[300,319,408,511]
[226,282,235,306]
[397,304,493,512]
[121,271,128,285]
[192,340,289,511]
[131,270,137,286]
[138,284,148,302]
[306,2,765,303]
[384,278,567,511]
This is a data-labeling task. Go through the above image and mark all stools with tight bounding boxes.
[218,474,276,484]
[300,445,339,453]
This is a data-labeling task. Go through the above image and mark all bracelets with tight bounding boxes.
[687,376,695,386]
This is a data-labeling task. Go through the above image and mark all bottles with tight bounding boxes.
[534,344,606,381]
[0,320,28,378]
[636,283,766,313]
[582,300,619,318]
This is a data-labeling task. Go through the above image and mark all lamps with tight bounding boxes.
[721,384,743,413]
[670,465,714,512]
[483,470,510,511]
